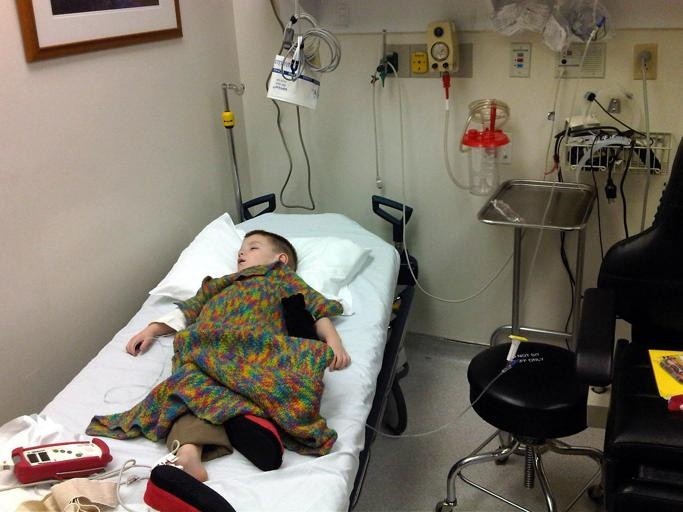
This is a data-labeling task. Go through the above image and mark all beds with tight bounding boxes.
[3,212,418,512]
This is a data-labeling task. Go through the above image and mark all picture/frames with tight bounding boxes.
[12,0,184,64]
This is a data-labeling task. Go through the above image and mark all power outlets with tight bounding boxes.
[633,43,658,80]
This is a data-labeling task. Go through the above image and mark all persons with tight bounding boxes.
[124,230,349,482]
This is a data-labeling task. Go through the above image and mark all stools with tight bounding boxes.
[434,342,604,512]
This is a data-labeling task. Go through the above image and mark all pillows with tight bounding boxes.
[146,213,373,318]
